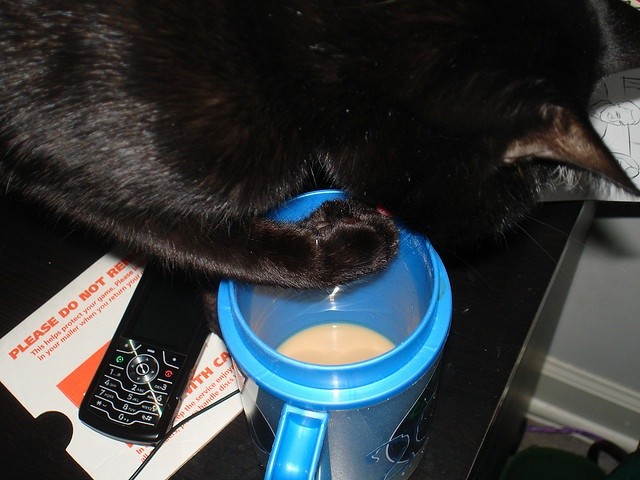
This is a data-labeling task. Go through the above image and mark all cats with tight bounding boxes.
[0,0,640,290]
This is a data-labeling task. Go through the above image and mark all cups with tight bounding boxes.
[216,189,453,480]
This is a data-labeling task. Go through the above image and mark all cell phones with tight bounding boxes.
[78,260,213,446]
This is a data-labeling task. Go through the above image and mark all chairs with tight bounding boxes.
[501,438,639,480]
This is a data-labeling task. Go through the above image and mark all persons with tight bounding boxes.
[581,74,640,141]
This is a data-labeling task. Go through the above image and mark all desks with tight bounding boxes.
[0,2,597,480]
